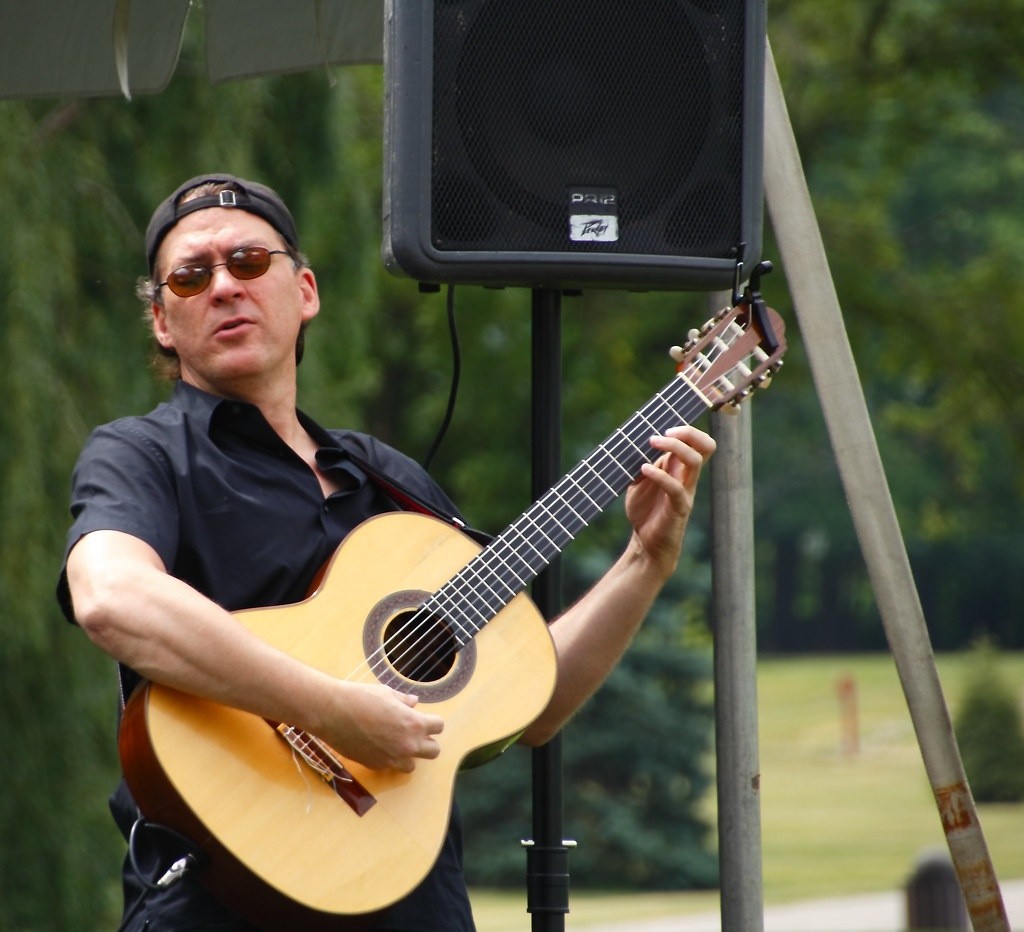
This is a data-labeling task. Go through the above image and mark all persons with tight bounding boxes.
[56,174,716,929]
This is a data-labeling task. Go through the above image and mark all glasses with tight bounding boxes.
[154,247,290,298]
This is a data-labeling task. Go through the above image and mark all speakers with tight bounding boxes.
[379,0,769,297]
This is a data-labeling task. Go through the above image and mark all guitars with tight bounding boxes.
[118,300,791,932]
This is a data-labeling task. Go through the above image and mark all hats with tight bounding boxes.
[146,174,297,276]
